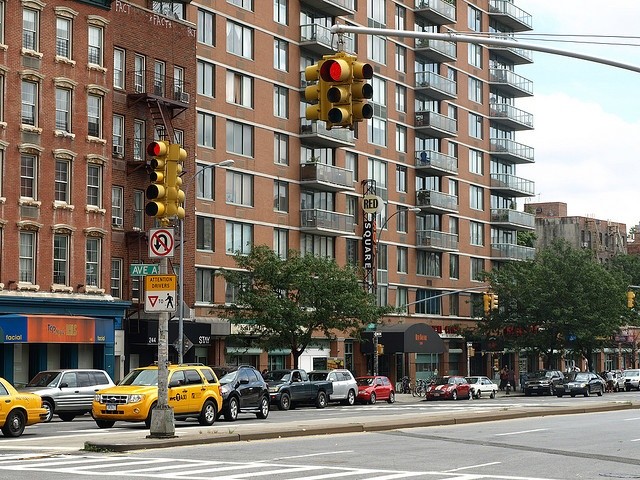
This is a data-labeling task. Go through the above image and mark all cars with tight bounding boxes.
[0,378,48,437]
[426,376,471,400]
[556,372,606,397]
[465,376,498,398]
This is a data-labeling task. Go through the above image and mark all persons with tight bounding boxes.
[313,375,320,381]
[499,365,516,391]
[261,369,270,380]
[292,372,301,382]
[607,369,615,393]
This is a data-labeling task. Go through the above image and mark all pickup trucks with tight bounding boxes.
[263,369,332,411]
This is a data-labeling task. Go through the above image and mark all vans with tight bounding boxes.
[19,369,116,421]
[623,369,640,391]
[307,369,358,405]
[354,376,395,404]
[606,369,626,392]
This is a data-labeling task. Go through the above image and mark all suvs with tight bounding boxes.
[206,364,270,421]
[524,370,564,395]
[91,363,223,428]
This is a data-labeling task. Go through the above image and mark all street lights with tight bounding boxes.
[374,207,421,376]
[178,159,235,364]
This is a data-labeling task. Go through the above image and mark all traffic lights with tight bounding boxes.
[145,141,169,221]
[320,52,356,130]
[484,295,491,316]
[169,144,187,218]
[491,293,499,311]
[305,60,321,121]
[628,291,635,308]
[351,63,373,122]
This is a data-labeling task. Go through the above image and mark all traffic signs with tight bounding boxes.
[143,274,177,313]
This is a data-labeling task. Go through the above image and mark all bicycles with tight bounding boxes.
[397,378,410,394]
[416,379,426,397]
